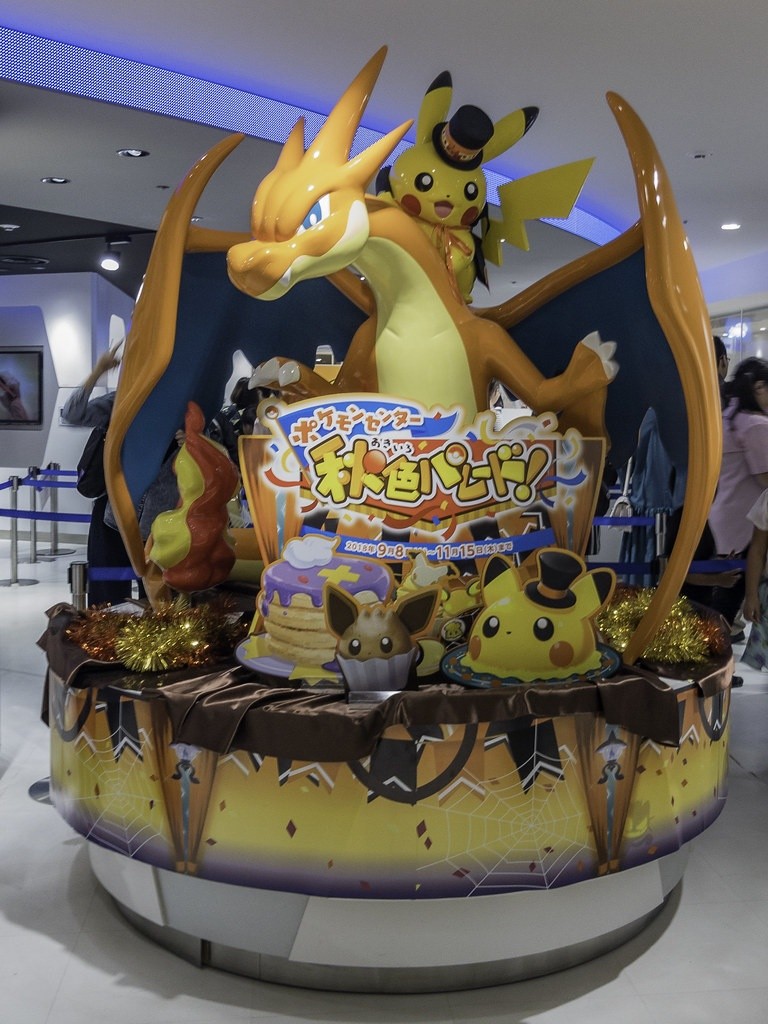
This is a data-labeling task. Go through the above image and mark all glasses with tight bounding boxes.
[719,355,731,362]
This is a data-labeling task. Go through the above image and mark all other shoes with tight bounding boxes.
[730,676,745,689]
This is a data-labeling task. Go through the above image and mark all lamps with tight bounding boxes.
[99,235,133,271]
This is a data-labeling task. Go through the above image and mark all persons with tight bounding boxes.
[60,340,186,609]
[682,335,732,604]
[707,357,767,686]
[744,489,768,672]
[585,406,680,591]
